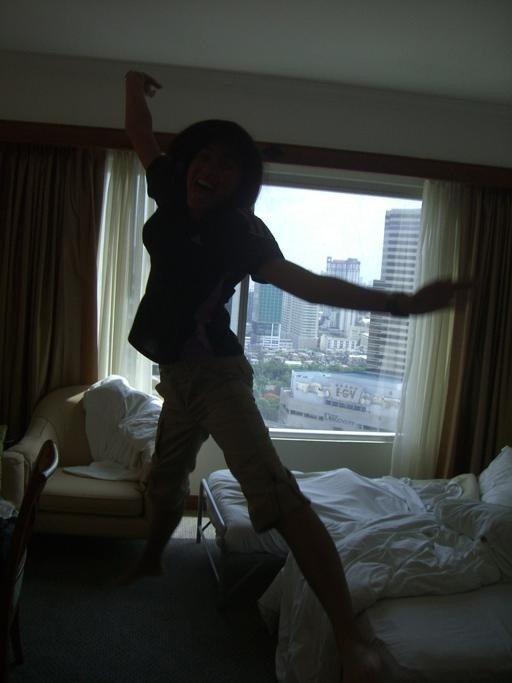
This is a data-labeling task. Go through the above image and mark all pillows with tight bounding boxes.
[478,445,511,507]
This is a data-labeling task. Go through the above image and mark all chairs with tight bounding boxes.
[0,439,60,682]
[0,383,153,540]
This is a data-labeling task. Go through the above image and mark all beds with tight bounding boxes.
[197,445,512,611]
[353,583,510,682]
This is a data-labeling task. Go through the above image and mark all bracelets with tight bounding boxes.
[391,291,409,317]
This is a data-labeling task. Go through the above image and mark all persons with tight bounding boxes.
[124,70,461,683]
